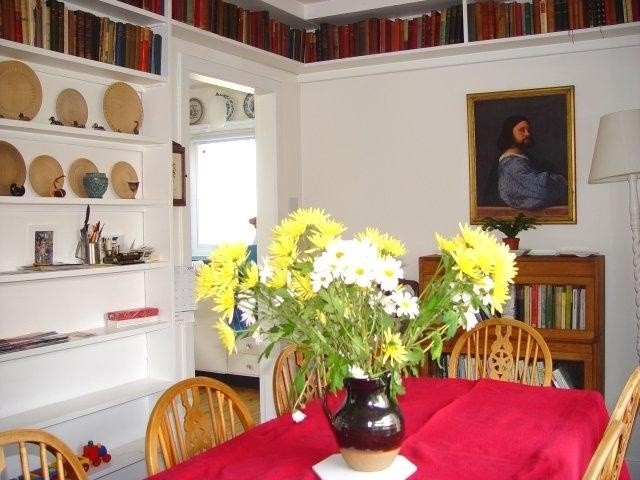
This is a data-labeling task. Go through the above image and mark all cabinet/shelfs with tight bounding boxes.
[418,251,605,388]
[0,0,179,480]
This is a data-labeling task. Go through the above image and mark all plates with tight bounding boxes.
[1,59,145,199]
[510,249,599,257]
[191,93,256,122]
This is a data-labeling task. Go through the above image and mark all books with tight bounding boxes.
[432,281,587,390]
[1,1,162,75]
[468,1,639,42]
[172,1,463,64]
[1,330,70,353]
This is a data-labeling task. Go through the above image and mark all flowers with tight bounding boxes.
[193,206,519,424]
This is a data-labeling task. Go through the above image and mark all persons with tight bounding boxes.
[497,115,568,210]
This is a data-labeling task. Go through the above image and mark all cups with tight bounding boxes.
[80,242,102,266]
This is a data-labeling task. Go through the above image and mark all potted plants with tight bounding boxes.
[483,212,541,258]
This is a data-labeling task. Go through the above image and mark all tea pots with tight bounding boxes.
[321,375,404,471]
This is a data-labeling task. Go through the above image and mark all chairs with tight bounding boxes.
[581,365,640,480]
[272,342,328,417]
[0,430,90,479]
[144,377,257,478]
[448,317,553,390]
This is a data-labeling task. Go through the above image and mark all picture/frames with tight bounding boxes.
[467,84,578,226]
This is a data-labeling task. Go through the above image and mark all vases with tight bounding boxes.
[333,376,404,471]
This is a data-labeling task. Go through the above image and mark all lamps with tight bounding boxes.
[587,108,640,366]
